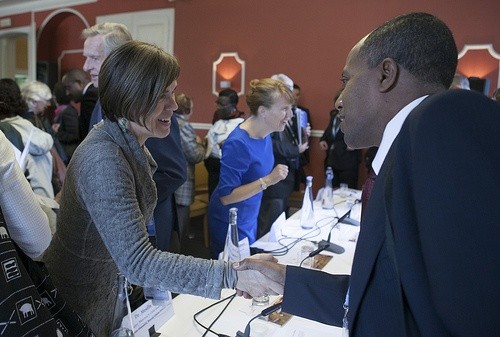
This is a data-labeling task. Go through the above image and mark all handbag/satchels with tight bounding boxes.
[0,206,96,337]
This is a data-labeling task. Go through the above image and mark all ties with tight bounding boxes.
[360,171,377,226]
[291,107,300,145]
[334,117,341,131]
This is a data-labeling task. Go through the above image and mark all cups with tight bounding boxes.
[339,183,349,197]
[301,244,315,269]
[251,295,270,306]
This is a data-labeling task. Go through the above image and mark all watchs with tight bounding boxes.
[259,178,266,190]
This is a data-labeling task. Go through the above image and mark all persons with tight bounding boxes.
[234,12,500,337]
[33,42,284,337]
[0,22,360,337]
[207,79,292,260]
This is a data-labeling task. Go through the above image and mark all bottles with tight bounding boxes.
[300,176,315,229]
[222,207,241,262]
[322,167,334,209]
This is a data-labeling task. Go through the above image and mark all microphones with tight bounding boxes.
[318,209,352,253]
[244,303,281,337]
[299,243,330,267]
[342,199,362,226]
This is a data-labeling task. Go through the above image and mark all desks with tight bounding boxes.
[151,188,361,337]
